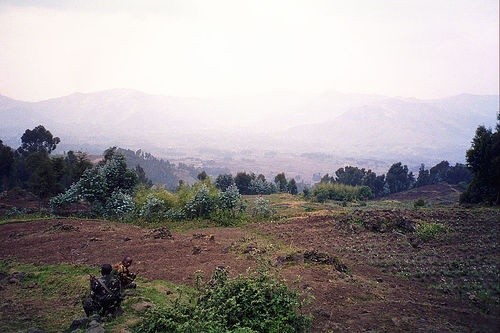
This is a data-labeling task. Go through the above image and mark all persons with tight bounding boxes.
[83,263,122,319]
[113,255,141,303]
